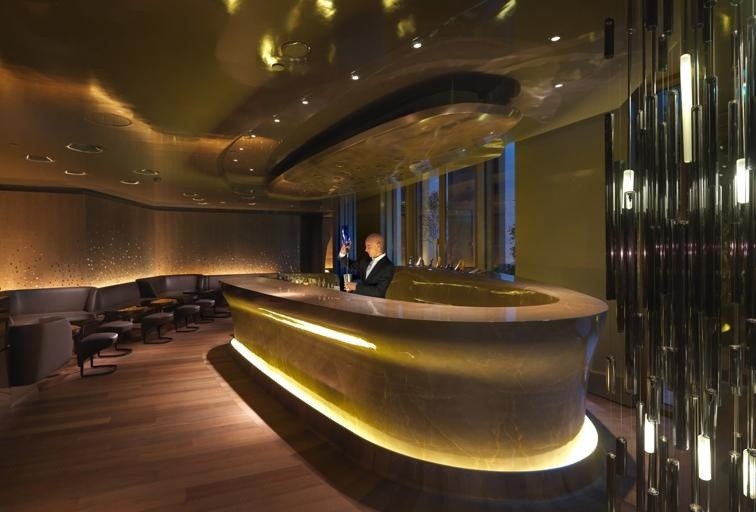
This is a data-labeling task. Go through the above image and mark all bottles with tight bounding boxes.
[342,226,351,254]
[277,272,335,289]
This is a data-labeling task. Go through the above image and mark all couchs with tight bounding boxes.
[0,273,228,389]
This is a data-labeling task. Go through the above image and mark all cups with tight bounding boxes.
[344,274,353,290]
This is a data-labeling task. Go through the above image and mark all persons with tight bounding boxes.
[337,233,394,299]
